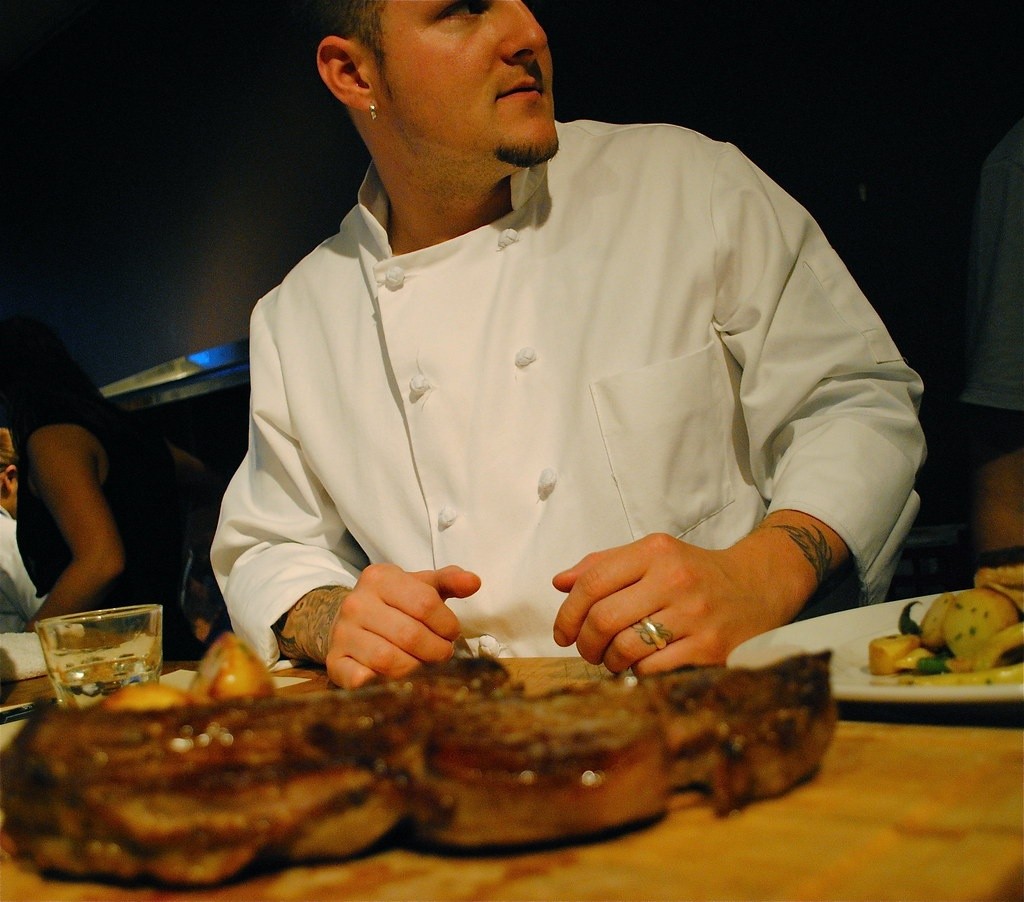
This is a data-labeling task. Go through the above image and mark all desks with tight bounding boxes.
[0,663,1024,902]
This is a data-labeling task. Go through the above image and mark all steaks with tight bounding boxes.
[0,654,512,885]
[391,649,838,851]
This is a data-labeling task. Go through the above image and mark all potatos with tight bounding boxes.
[868,586,1019,677]
[105,629,272,709]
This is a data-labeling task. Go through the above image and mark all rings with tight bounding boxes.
[640,616,668,650]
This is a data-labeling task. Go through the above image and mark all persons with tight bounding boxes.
[949,448,1024,592]
[0,316,222,665]
[0,428,49,632]
[212,0,929,691]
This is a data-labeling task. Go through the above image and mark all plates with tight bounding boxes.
[725,587,1024,700]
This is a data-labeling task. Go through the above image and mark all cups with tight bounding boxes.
[35,603,163,713]
